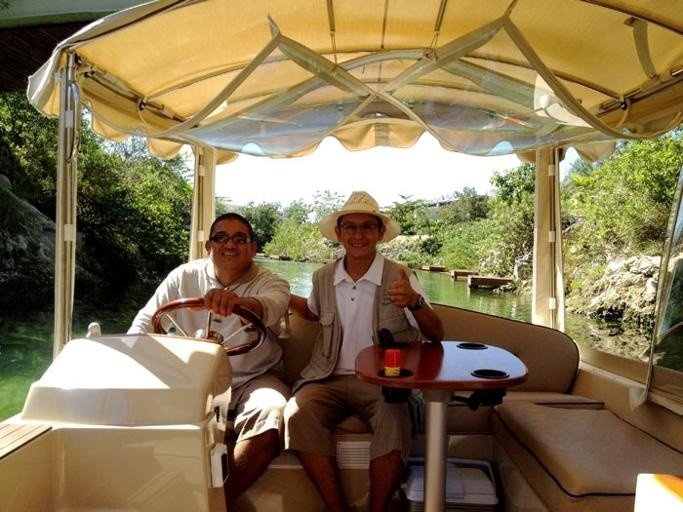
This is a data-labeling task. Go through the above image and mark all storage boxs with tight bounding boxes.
[405,466,498,512]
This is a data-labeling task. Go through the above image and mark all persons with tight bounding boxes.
[122,208,294,504]
[277,186,448,511]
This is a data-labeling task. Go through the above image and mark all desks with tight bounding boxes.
[354,335,529,512]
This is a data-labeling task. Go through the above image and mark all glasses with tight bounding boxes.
[334,220,382,237]
[210,230,252,247]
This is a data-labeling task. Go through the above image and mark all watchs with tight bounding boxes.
[407,288,425,312]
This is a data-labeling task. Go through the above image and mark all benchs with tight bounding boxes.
[104,292,606,465]
[493,400,683,511]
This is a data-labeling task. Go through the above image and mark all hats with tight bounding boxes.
[317,189,402,245]
